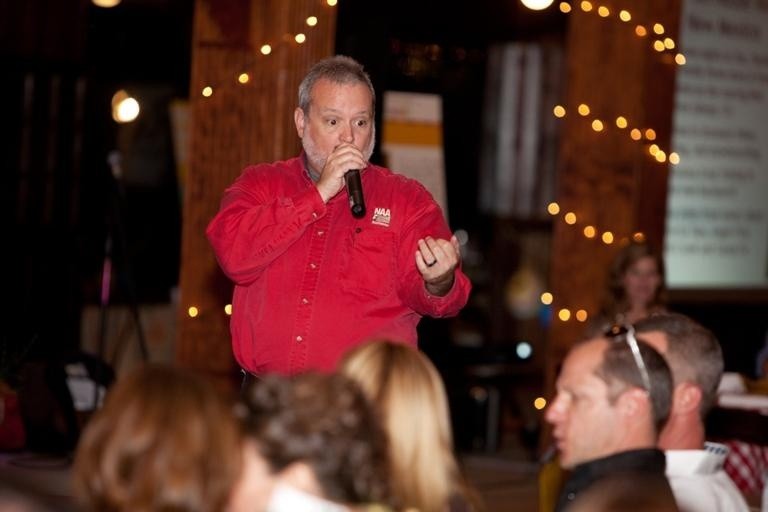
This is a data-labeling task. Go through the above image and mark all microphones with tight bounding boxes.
[343,168,366,219]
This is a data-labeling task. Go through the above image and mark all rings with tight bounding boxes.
[428,260,437,267]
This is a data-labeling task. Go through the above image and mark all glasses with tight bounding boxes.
[603,321,652,391]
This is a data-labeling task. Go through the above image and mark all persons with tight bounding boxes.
[630,311,753,512]
[244,371,417,511]
[597,243,669,333]
[344,338,488,512]
[72,365,348,511]
[205,53,475,396]
[543,321,683,511]
